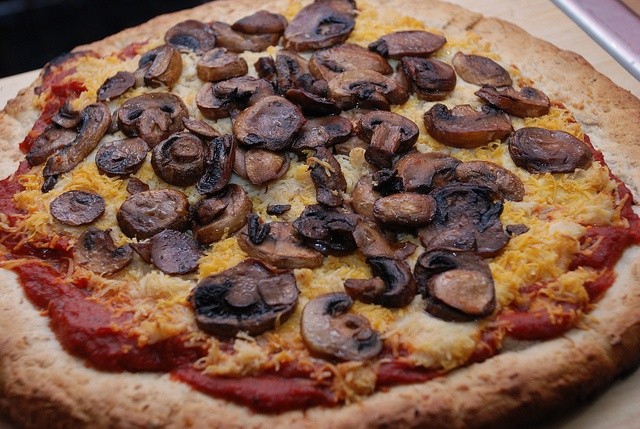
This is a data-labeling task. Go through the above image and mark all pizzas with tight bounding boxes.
[1,1,640,429]
